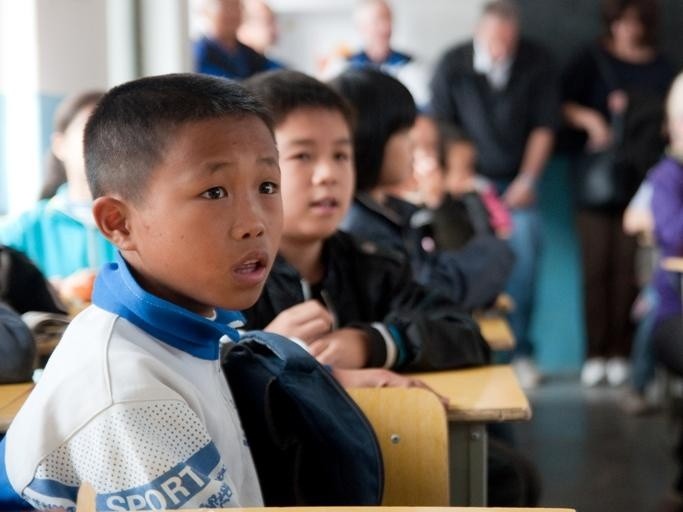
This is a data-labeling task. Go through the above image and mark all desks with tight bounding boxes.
[0,287,531,510]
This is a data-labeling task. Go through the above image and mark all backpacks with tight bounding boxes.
[221,330,383,508]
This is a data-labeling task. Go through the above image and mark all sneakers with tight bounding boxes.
[582,357,659,417]
[510,358,544,389]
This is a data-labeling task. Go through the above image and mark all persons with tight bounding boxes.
[0,69,451,511]
[0,1,682,419]
[241,68,544,508]
